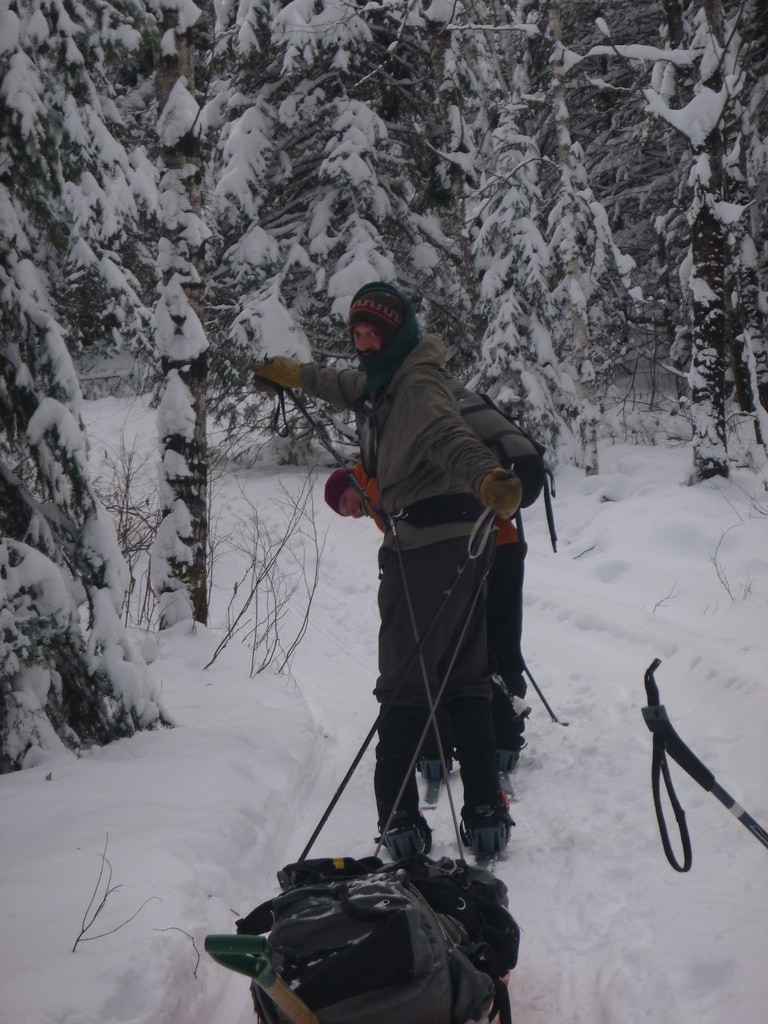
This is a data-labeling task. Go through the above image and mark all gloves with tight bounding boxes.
[478,468,522,519]
[248,356,306,392]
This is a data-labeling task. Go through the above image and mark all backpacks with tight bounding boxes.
[374,364,546,526]
[205,857,519,1024]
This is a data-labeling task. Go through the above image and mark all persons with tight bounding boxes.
[249,281,521,863]
[324,463,526,780]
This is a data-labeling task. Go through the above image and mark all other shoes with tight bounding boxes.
[410,725,456,780]
[375,776,432,861]
[459,772,515,855]
[490,705,531,771]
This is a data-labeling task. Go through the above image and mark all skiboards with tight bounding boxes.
[388,747,519,877]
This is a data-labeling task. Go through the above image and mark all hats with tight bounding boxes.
[324,467,355,516]
[347,291,405,336]
